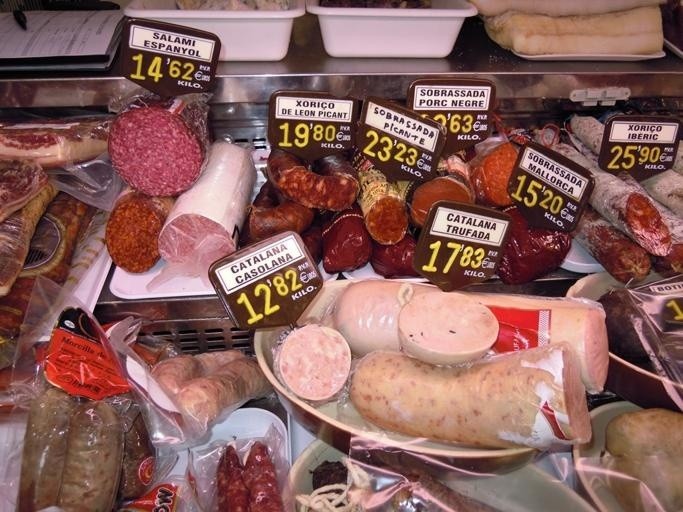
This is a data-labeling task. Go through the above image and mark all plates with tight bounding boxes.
[283,435,600,511]
[572,400,645,512]
[251,277,534,458]
[564,271,682,388]
